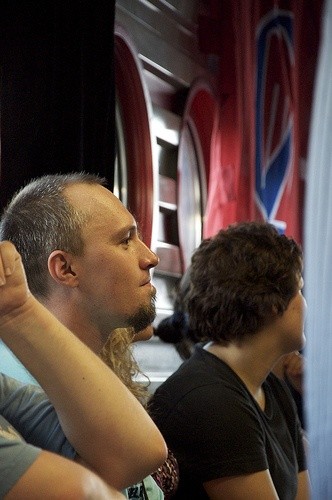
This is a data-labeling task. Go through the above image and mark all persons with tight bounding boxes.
[0,240,169,500]
[0,171,165,500]
[143,218,312,500]
